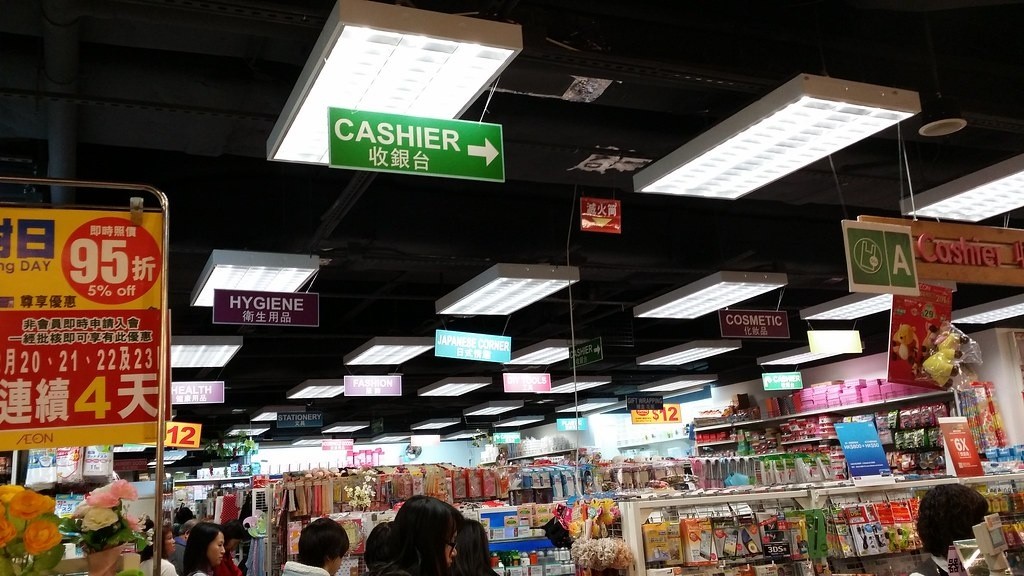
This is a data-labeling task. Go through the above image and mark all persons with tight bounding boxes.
[360,495,501,576]
[281,518,350,576]
[908,483,990,576]
[138,507,245,576]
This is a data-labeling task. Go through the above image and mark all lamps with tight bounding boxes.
[951,293,1024,324]
[170,335,243,367]
[463,401,525,415]
[371,432,416,441]
[800,292,892,320]
[632,271,788,320]
[536,376,611,393]
[435,263,580,315]
[285,378,344,398]
[190,250,320,308]
[417,377,492,396]
[223,424,270,436]
[266,0,522,166]
[502,339,589,365]
[899,152,1024,223]
[492,415,545,426]
[636,340,742,365]
[633,73,921,200]
[343,335,435,364]
[250,406,306,421]
[636,374,718,392]
[444,429,488,439]
[756,340,866,365]
[409,418,460,429]
[321,421,370,433]
[554,398,619,412]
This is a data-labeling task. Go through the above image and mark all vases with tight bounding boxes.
[88,542,128,576]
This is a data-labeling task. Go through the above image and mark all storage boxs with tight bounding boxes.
[696,377,937,480]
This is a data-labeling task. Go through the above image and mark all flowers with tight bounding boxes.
[0,484,66,576]
[340,471,377,510]
[60,479,149,554]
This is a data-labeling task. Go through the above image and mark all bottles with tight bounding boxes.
[489,547,572,568]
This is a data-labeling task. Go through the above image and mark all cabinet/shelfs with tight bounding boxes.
[615,434,693,468]
[481,447,585,466]
[693,387,962,475]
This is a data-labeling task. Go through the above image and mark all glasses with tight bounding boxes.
[446,539,458,549]
